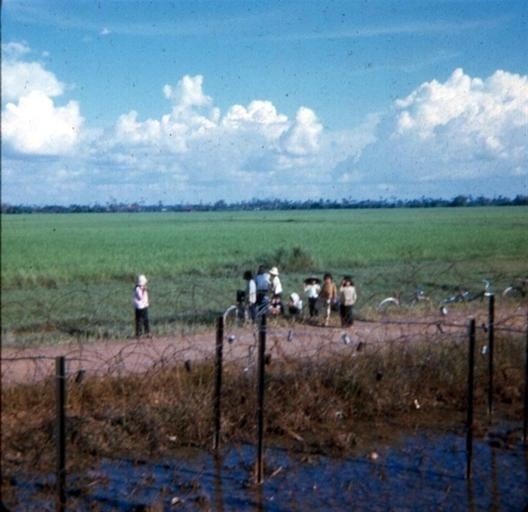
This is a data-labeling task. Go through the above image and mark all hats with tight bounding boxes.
[136,274,148,286]
[269,266,279,275]
[303,275,322,285]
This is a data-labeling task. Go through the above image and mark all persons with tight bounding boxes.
[286,291,302,316]
[337,276,357,329]
[303,278,322,318]
[253,264,270,326]
[320,273,336,327]
[131,273,153,341]
[237,268,257,325]
[267,266,285,316]
